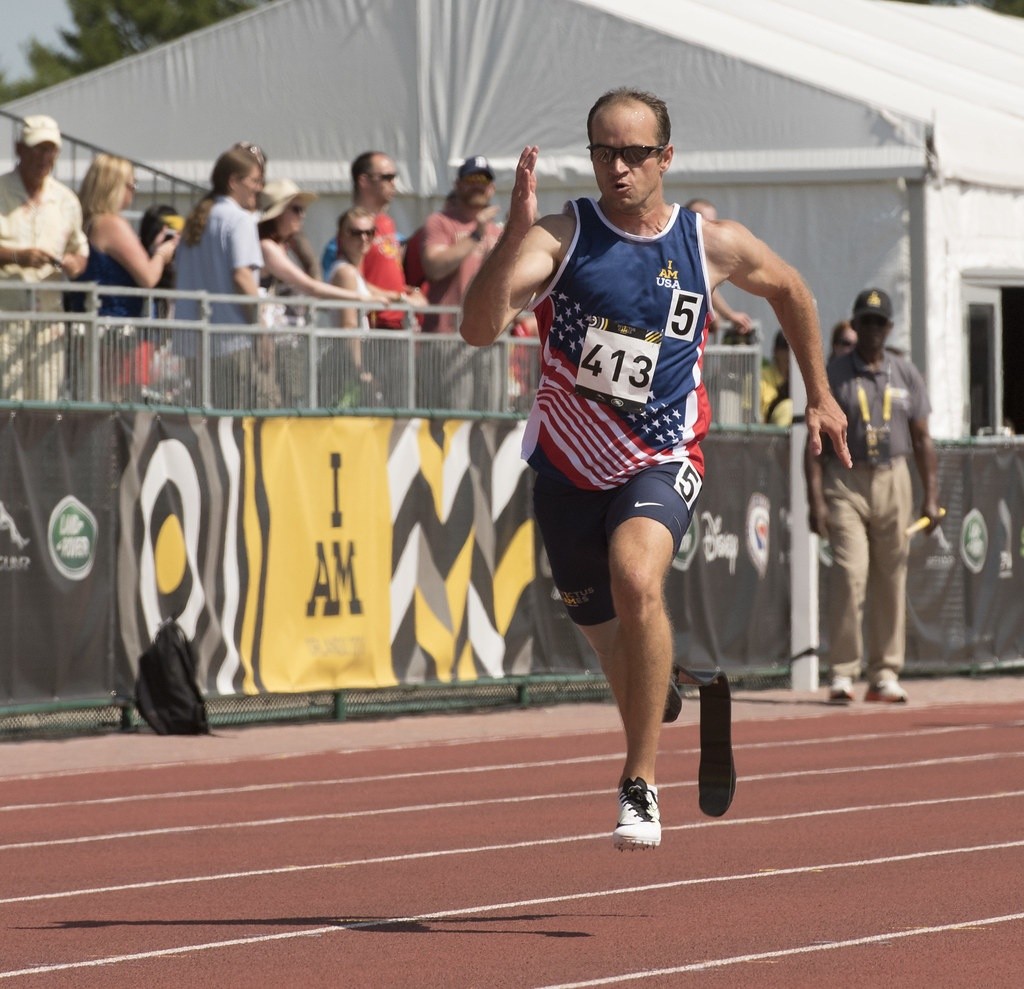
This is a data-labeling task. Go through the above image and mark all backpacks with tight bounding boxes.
[133,621,212,740]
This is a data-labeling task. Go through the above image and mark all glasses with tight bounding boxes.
[368,170,394,182]
[587,142,663,165]
[287,203,303,212]
[350,228,375,237]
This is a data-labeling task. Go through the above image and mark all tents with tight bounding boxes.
[3,1,1024,441]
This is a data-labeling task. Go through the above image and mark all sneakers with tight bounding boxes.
[611,776,662,854]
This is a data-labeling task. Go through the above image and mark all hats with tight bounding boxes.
[255,177,318,223]
[851,288,892,328]
[15,113,62,151]
[458,154,496,183]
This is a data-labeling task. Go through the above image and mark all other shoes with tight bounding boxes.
[826,675,855,705]
[865,677,908,704]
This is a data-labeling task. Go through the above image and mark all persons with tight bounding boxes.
[457,88,855,852]
[687,199,856,431]
[1,111,545,415]
[802,287,940,706]
